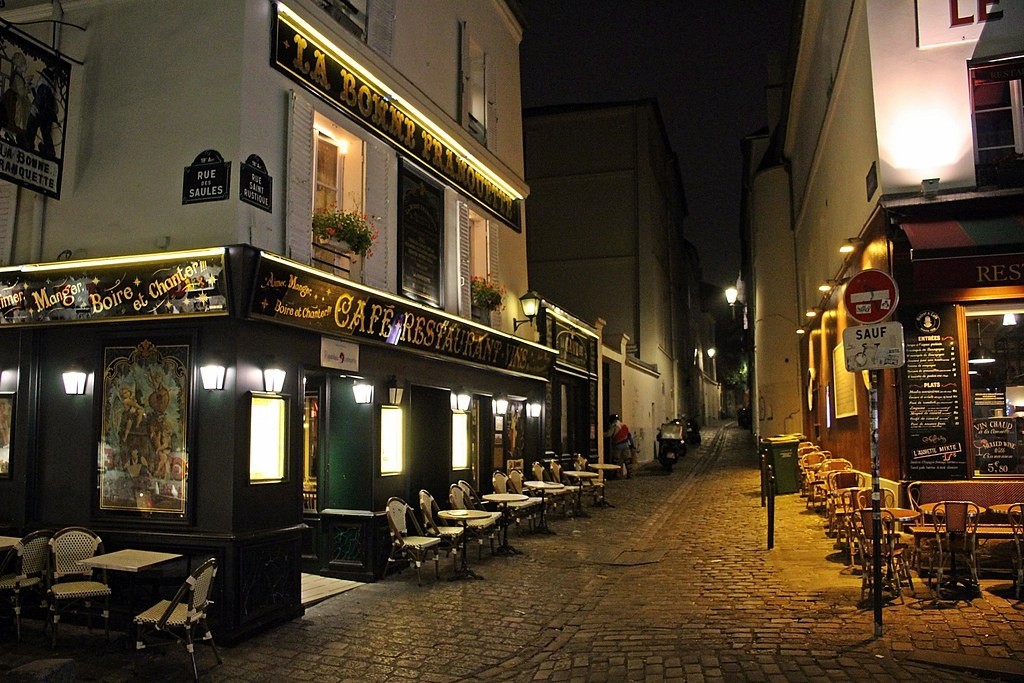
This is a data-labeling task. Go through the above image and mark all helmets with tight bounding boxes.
[608,414,619,424]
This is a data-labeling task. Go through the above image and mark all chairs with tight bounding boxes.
[0,527,222,683]
[797,441,1024,606]
[386,454,604,587]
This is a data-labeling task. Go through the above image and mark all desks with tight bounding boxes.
[0,536,23,548]
[989,503,1024,517]
[919,503,986,522]
[437,462,622,580]
[877,508,921,586]
[73,549,183,657]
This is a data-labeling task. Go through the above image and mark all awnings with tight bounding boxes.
[898,210,1024,261]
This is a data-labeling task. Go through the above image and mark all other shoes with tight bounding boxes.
[625,475,632,480]
[613,476,621,480]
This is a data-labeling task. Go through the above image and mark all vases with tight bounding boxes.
[313,227,355,257]
[472,296,491,308]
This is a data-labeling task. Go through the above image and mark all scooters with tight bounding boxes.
[656,412,702,471]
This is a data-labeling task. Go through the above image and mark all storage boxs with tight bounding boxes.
[661,424,683,439]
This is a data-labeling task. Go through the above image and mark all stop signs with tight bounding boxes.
[843,269,899,325]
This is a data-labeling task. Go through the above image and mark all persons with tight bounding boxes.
[0,53,62,157]
[603,414,636,478]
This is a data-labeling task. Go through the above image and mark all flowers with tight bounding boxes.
[470,272,506,310]
[313,204,380,255]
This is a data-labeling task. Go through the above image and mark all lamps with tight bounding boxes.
[0,369,17,394]
[819,278,841,291]
[263,368,542,419]
[840,237,864,252]
[806,306,825,317]
[795,325,813,334]
[62,371,87,394]
[202,365,226,391]
[514,290,541,331]
[968,313,1017,375]
[921,177,941,200]
[725,287,756,307]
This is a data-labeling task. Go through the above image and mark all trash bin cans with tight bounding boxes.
[762,436,800,495]
